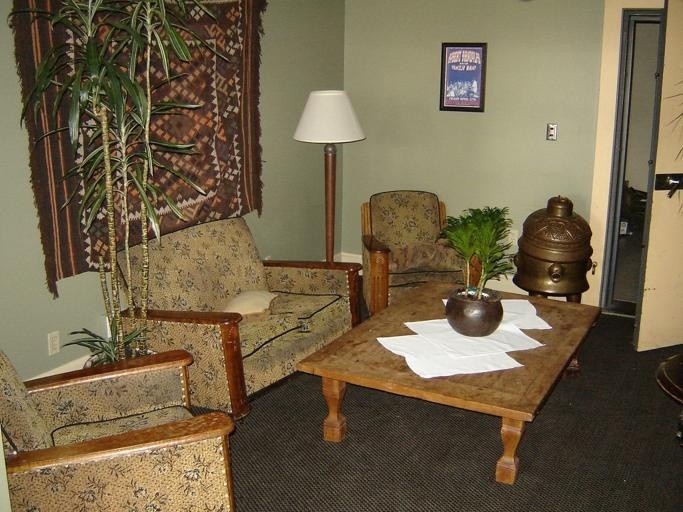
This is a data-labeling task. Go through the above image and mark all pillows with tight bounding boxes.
[224,290,278,314]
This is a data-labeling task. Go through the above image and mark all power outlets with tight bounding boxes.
[48,331,60,356]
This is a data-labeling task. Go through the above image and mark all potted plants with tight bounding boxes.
[5,0,232,370]
[434,205,519,338]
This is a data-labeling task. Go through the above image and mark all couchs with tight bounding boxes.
[117,216,363,420]
[361,190,467,317]
[1,350,235,512]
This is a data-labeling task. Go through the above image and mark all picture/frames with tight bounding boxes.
[439,42,487,113]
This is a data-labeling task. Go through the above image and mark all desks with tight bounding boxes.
[630,196,651,213]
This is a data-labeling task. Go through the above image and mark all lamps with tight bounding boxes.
[292,89,367,262]
